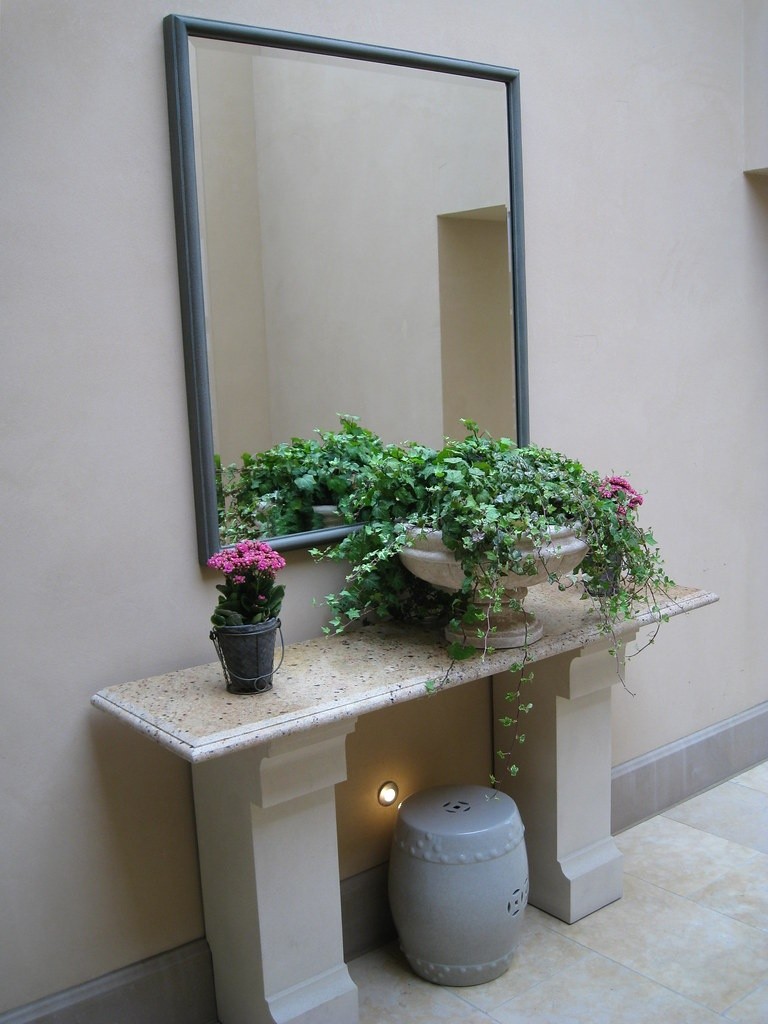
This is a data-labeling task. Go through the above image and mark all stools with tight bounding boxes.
[389,784,529,988]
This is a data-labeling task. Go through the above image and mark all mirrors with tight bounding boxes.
[164,14,531,573]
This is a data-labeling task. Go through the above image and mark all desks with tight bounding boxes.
[90,574,720,1024]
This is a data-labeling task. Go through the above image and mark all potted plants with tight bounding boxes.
[220,414,436,545]
[307,419,689,803]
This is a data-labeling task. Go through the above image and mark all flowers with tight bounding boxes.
[210,541,287,629]
[598,475,646,523]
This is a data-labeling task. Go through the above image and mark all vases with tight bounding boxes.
[583,549,623,597]
[211,616,281,694]
